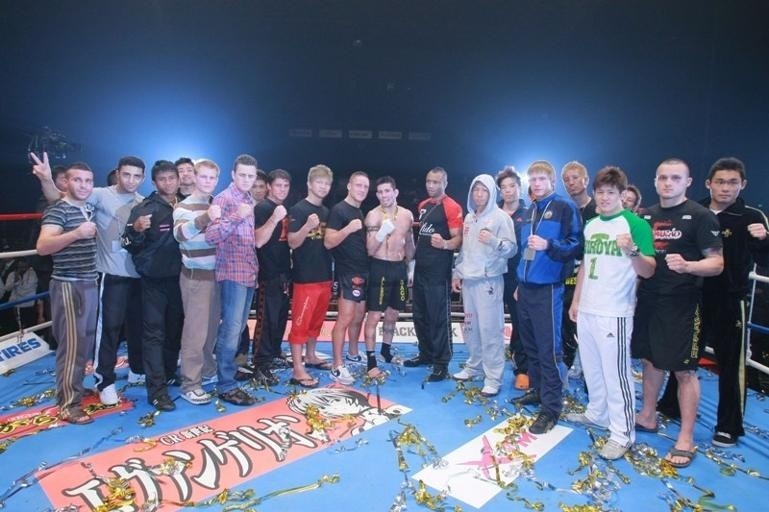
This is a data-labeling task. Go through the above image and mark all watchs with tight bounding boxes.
[442,239,448,249]
[628,245,640,257]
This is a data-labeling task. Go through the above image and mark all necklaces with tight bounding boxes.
[418,194,447,227]
[381,205,399,220]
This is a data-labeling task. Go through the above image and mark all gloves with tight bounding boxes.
[377,219,395,243]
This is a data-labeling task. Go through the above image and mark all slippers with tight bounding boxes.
[290,377,319,387]
[634,421,657,433]
[305,362,330,369]
[665,445,697,467]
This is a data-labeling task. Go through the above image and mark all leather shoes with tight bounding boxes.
[515,373,529,390]
[428,370,446,382]
[452,369,484,381]
[530,415,553,434]
[404,357,423,367]
[600,439,627,460]
[564,413,587,423]
[219,357,294,405]
[511,391,536,405]
[480,388,498,397]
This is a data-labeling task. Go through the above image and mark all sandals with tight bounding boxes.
[68,408,93,425]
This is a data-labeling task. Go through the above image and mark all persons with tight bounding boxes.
[28,165,67,350]
[234,170,287,365]
[452,174,518,397]
[512,159,768,470]
[121,159,184,411]
[30,151,146,405]
[363,176,416,380]
[497,165,527,360]
[404,166,464,382]
[1,193,45,337]
[324,171,368,383]
[175,158,214,203]
[205,154,260,406]
[172,160,219,404]
[108,170,117,186]
[288,164,332,387]
[37,160,99,423]
[250,166,294,386]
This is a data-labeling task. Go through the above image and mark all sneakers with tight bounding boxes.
[128,370,147,384]
[100,384,118,405]
[329,365,355,385]
[148,395,175,411]
[202,375,218,385]
[167,376,181,386]
[712,431,737,446]
[346,351,368,363]
[182,389,212,405]
